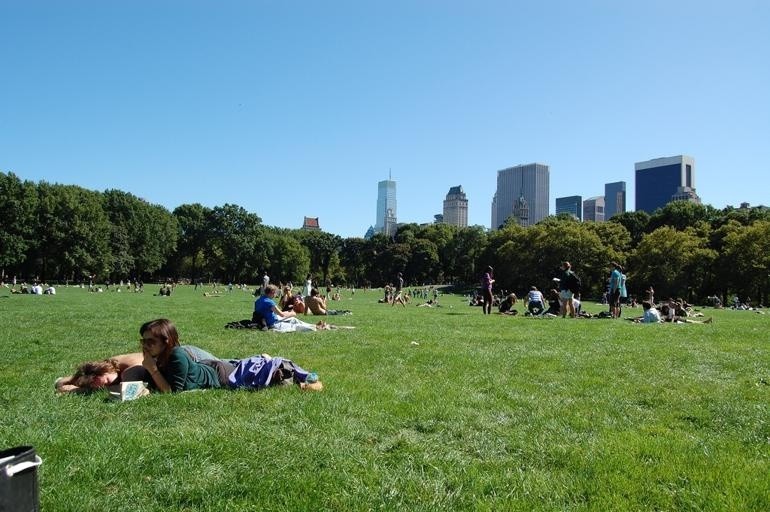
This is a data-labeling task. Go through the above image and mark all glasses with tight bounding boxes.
[140,338,156,345]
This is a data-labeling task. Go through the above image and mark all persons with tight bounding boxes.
[259,261,769,323]
[1,274,247,296]
[253,284,326,332]
[52,345,241,394]
[253,286,322,326]
[139,318,323,395]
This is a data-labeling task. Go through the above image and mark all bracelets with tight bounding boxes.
[151,368,159,377]
